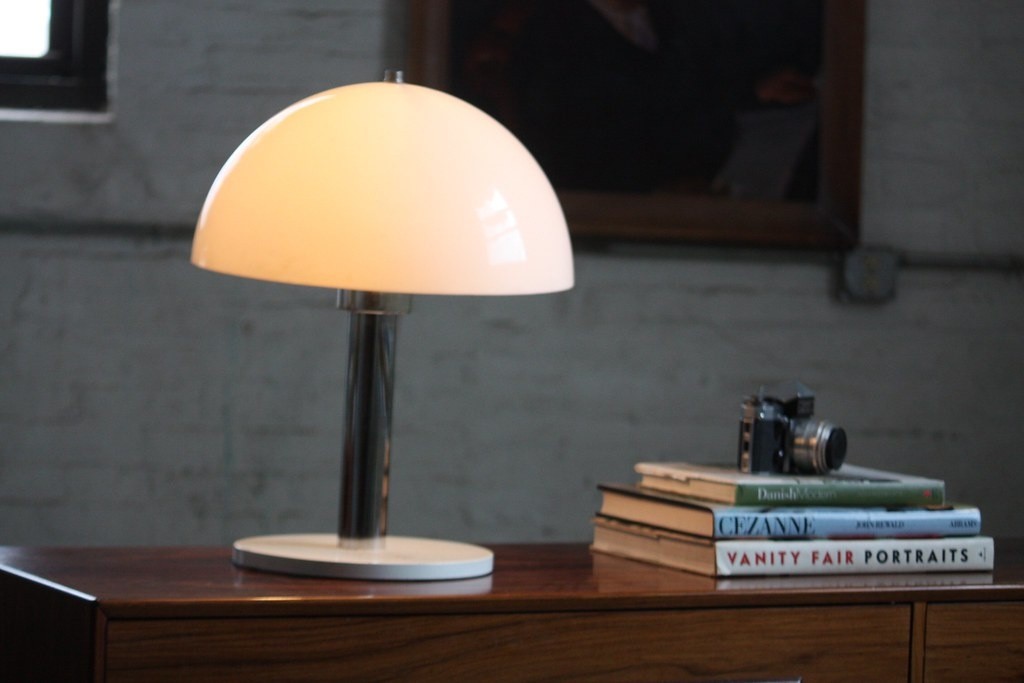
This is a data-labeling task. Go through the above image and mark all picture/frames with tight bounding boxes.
[410,0,866,247]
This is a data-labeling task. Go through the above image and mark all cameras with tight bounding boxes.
[737,394,849,477]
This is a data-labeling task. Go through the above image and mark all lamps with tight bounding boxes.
[190,68,577,580]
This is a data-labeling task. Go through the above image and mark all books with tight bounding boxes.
[590,463,994,578]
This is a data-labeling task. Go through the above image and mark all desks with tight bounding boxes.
[0,541,1024,683]
